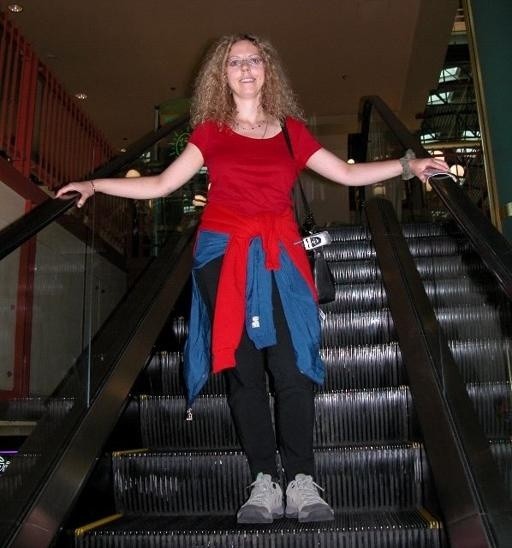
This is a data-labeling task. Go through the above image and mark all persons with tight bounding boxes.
[56,30,452,523]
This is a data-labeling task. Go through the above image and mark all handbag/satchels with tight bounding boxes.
[298,220,335,304]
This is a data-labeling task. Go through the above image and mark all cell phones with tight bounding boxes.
[302,231,332,251]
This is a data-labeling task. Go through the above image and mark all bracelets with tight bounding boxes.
[398,154,416,182]
[88,179,97,194]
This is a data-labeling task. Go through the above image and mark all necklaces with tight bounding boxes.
[228,114,269,133]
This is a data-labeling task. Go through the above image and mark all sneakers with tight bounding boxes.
[236,473,284,523]
[284,474,336,524]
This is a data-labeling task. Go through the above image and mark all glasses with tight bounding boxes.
[226,53,265,69]
